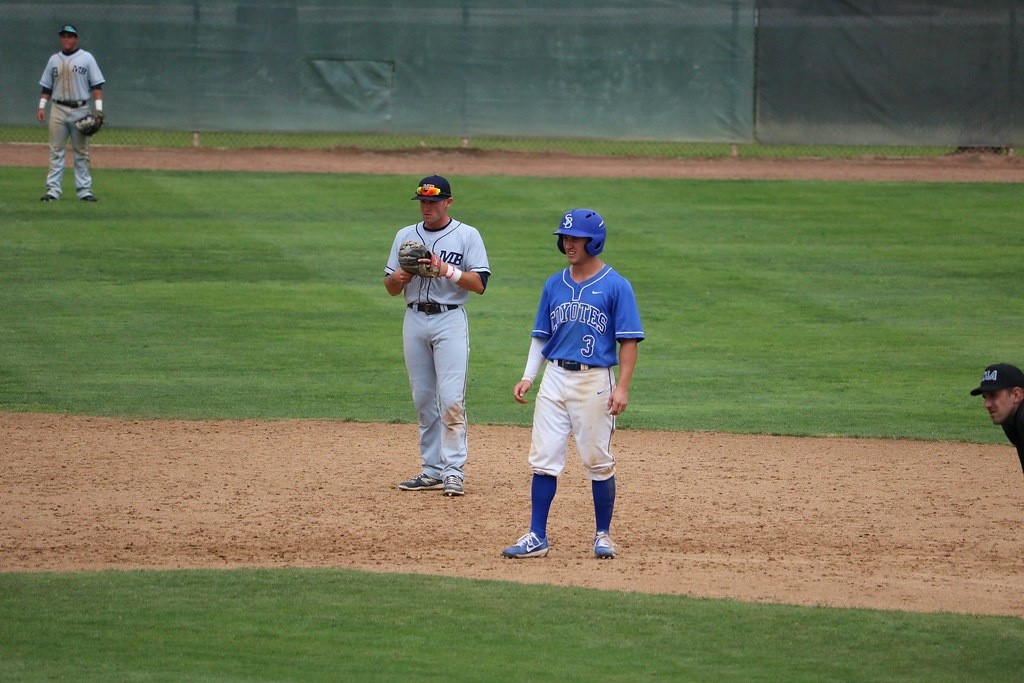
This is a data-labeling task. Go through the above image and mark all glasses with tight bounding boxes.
[415,187,440,196]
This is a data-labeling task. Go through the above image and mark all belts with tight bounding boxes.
[408,303,458,316]
[53,99,87,109]
[550,358,598,371]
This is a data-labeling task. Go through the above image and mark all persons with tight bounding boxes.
[38,25,105,203]
[969,362,1024,474]
[501,207,645,560]
[383,176,491,496]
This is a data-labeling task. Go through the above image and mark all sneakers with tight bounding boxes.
[593,534,617,559]
[502,532,549,558]
[398,472,445,490]
[442,475,464,495]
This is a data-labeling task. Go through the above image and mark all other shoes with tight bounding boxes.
[41,195,57,202]
[82,195,97,203]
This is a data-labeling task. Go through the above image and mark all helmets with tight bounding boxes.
[552,209,607,258]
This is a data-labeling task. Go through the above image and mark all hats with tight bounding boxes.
[970,363,1024,396]
[58,23,79,35]
[411,174,453,202]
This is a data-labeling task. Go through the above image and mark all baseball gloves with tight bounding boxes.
[397,241,442,279]
[74,110,104,136]
